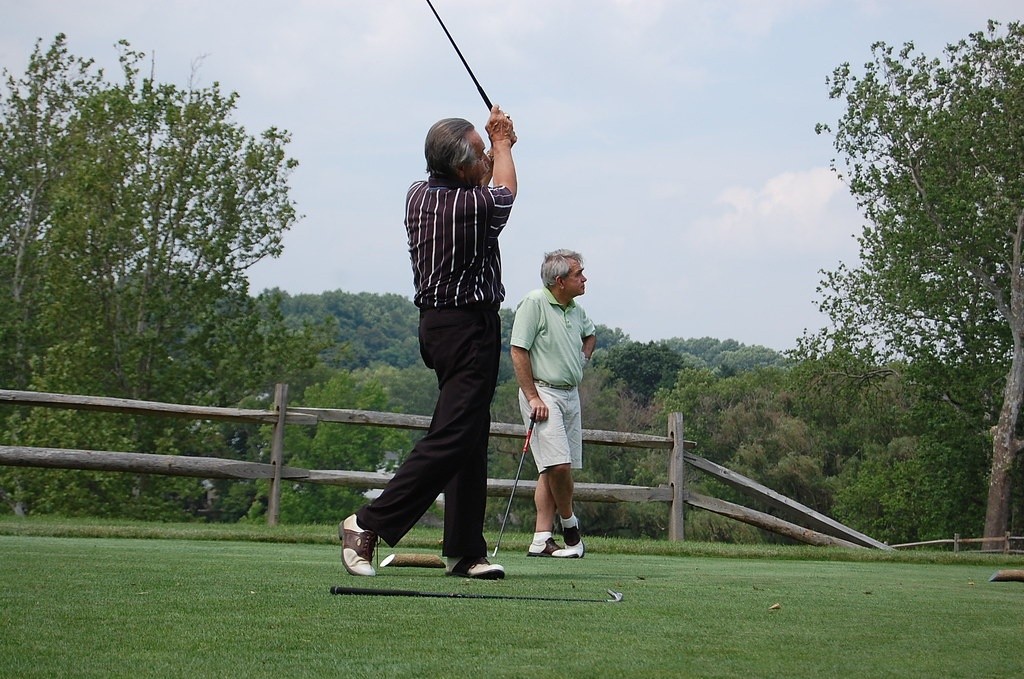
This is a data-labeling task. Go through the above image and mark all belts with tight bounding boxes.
[533,380,575,390]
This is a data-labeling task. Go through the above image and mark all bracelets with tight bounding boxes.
[486,150,495,163]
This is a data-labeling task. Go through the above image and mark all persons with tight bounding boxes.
[509,248,597,559]
[337,117,518,583]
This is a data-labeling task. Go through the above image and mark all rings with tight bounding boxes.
[504,113,510,119]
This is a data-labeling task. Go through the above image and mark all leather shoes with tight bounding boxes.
[338,513,378,577]
[445,553,505,578]
[526,538,562,559]
[560,515,585,558]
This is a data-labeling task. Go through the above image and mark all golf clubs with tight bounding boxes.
[486,418,536,558]
[330,584,622,603]
[426,0,494,111]
